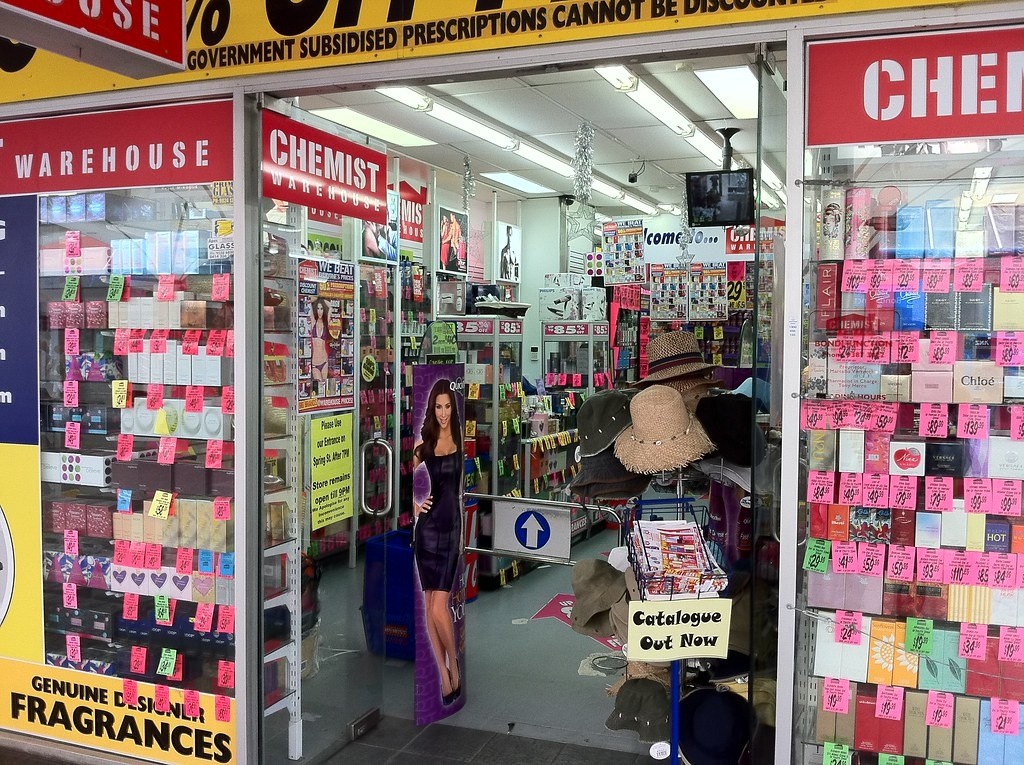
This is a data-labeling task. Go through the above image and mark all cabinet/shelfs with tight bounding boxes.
[35,183,304,761]
[542,320,610,421]
[437,314,526,581]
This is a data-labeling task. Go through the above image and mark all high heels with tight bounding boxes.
[440,658,460,705]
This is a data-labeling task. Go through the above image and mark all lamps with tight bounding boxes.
[425,93,519,152]
[731,150,787,210]
[685,122,724,167]
[593,65,637,92]
[511,136,575,178]
[617,188,660,217]
[592,173,622,200]
[625,74,696,136]
[375,85,430,110]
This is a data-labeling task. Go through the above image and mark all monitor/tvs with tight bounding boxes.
[686,168,755,227]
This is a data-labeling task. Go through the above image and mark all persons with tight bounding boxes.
[306,298,332,380]
[362,208,397,261]
[414,379,462,706]
[500,226,516,281]
[710,175,719,191]
[566,343,596,375]
[263,198,289,246]
[441,211,461,271]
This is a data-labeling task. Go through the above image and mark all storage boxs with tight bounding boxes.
[39,196,48,225]
[171,230,185,274]
[111,239,120,274]
[119,238,131,275]
[155,231,172,274]
[803,737,823,765]
[67,194,86,223]
[538,273,607,321]
[835,682,857,750]
[903,688,928,758]
[953,696,1024,765]
[854,683,879,752]
[183,229,210,275]
[806,177,1024,703]
[38,291,278,698]
[47,196,66,224]
[816,678,835,745]
[879,698,903,755]
[87,192,123,223]
[131,238,143,274]
[143,231,156,275]
[209,231,221,275]
[927,726,952,762]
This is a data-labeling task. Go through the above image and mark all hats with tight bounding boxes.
[570,559,756,765]
[575,330,781,498]
